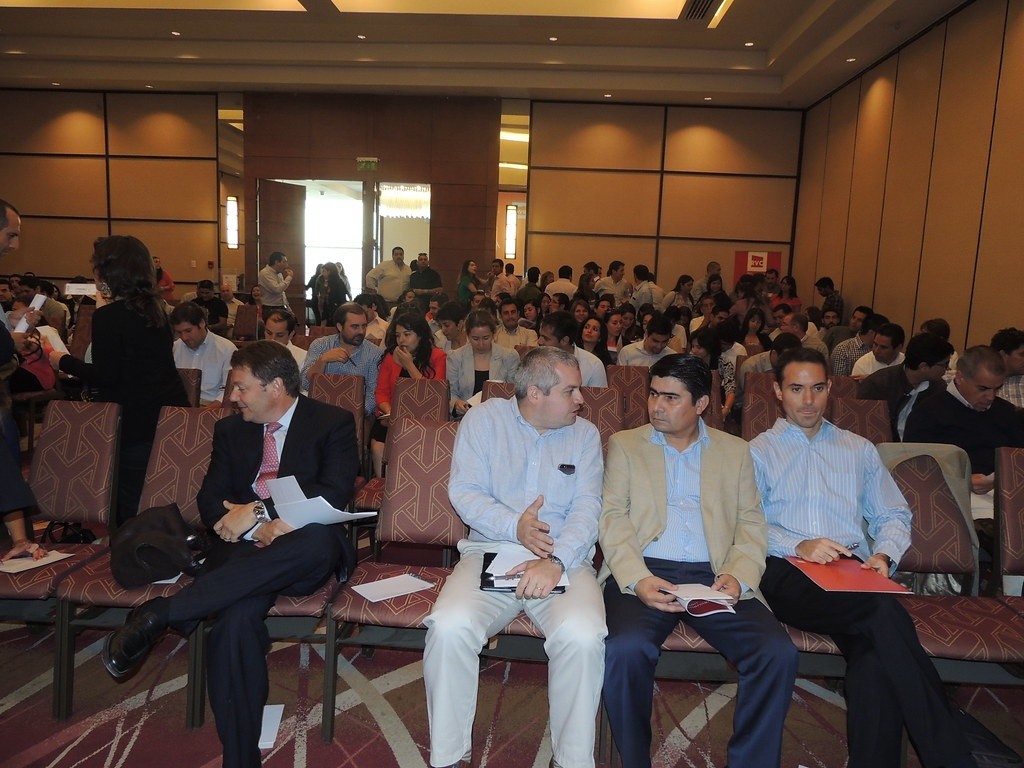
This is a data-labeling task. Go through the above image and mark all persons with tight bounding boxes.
[849,317,1024,594]
[0,400,48,562]
[102,340,359,768]
[0,205,904,478]
[748,346,977,768]
[599,353,798,768]
[423,347,609,768]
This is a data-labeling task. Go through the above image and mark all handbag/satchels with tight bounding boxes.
[111,502,217,589]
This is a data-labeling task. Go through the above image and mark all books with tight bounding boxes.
[480,553,570,594]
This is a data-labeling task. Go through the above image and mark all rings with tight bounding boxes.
[223,536,231,540]
[536,587,544,591]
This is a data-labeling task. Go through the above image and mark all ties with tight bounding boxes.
[255,422,283,500]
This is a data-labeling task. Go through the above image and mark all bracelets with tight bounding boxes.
[547,554,565,571]
[726,406,731,412]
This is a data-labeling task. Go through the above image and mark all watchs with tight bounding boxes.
[254,501,265,519]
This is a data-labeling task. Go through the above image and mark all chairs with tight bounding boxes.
[0,306,1024,745]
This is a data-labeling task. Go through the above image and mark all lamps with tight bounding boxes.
[225,196,239,249]
[505,205,519,259]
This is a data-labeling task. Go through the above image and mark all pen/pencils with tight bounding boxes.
[484,573,523,582]
[346,354,357,367]
[463,401,467,405]
[839,543,858,555]
[378,410,385,415]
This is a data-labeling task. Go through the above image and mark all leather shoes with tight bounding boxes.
[102,596,170,683]
